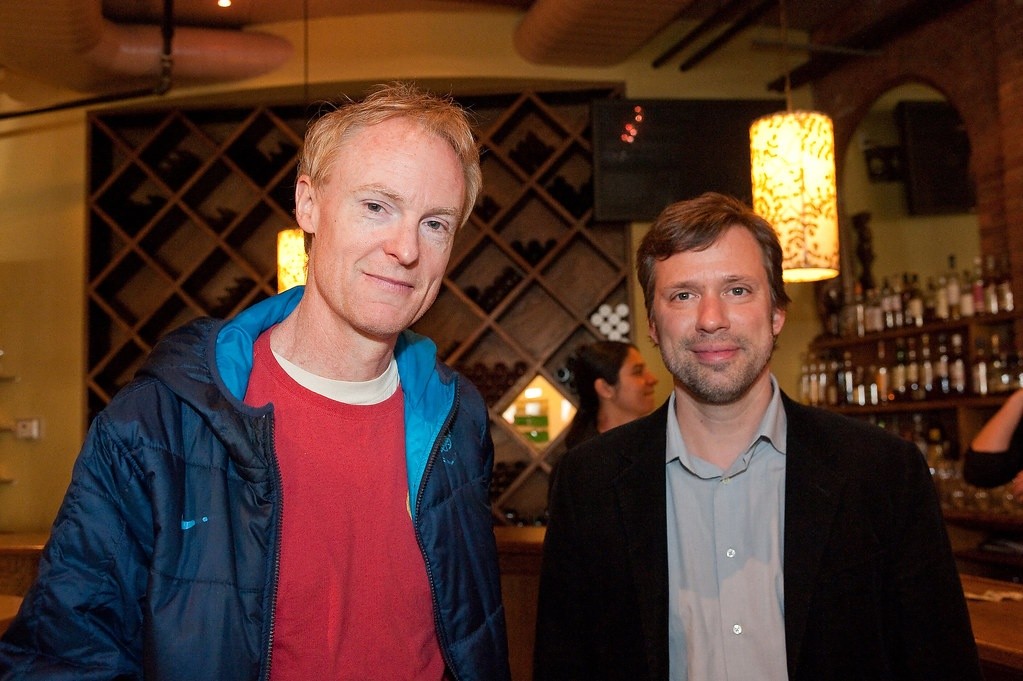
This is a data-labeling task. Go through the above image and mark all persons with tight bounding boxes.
[0,84,516,681]
[563,340,659,450]
[530,193,985,681]
[961,389,1023,489]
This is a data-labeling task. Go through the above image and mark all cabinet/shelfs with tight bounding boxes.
[804,313,1023,548]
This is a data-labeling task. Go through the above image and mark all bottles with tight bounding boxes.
[798,253,1023,512]
[87,115,630,529]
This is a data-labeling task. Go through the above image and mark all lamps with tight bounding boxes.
[274,0,310,292]
[749,0,842,285]
